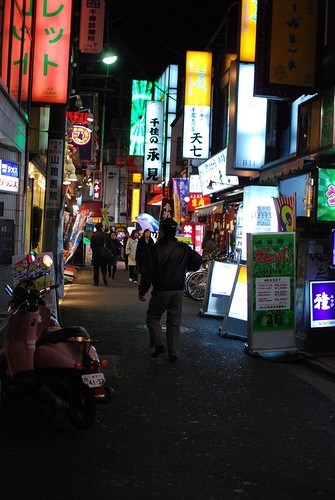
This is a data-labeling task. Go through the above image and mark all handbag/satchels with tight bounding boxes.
[101,246,113,261]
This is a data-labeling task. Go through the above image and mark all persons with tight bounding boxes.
[90,222,155,287]
[137,218,203,363]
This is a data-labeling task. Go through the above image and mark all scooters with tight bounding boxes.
[0,248,116,428]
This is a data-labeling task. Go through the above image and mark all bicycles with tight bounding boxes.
[185,249,241,301]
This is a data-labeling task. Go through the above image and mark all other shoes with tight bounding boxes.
[112,275,115,280]
[150,345,167,358]
[109,273,111,277]
[103,278,108,285]
[94,283,99,286]
[133,280,138,284]
[128,277,132,282]
[169,354,177,362]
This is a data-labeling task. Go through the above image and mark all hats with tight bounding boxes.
[160,218,177,231]
